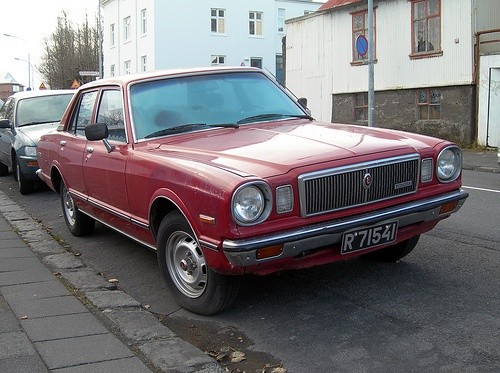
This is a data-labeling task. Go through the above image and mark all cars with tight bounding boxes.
[34,65,470,316]
[1,88,77,197]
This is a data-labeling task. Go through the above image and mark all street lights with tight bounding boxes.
[3,32,31,92]
